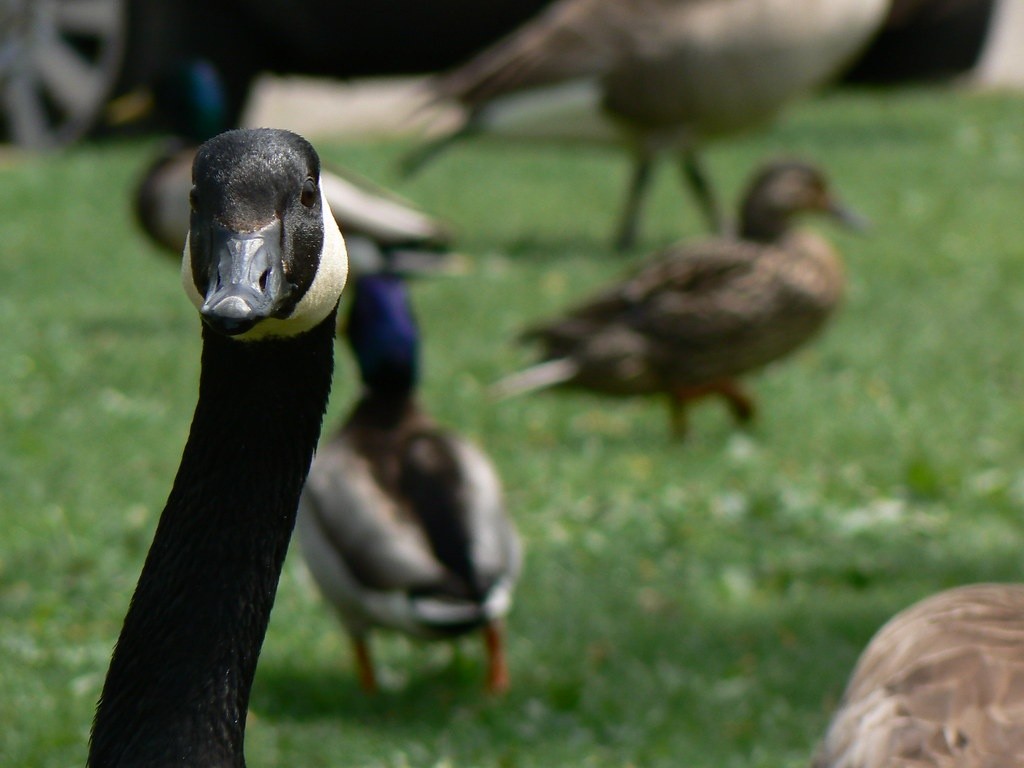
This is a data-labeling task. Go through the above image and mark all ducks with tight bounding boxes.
[84,1,1024,768]
